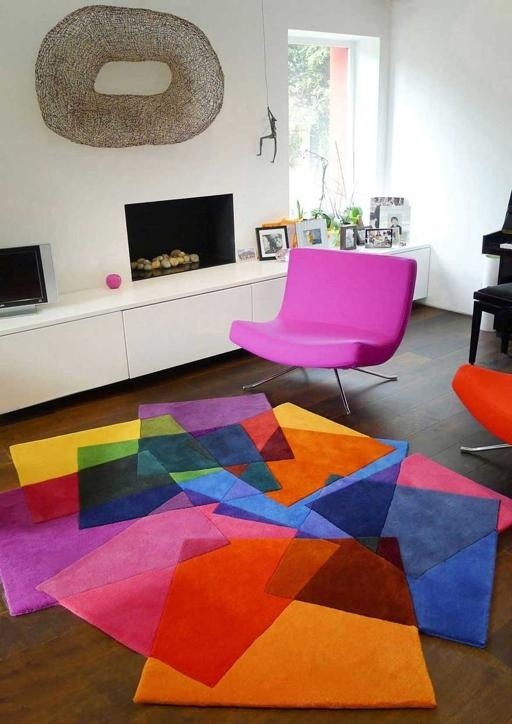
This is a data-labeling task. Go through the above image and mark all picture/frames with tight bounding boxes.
[255,206,411,260]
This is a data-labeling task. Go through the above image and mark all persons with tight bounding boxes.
[390,216,402,236]
[364,230,392,248]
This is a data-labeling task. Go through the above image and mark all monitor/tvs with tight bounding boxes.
[0,242,60,319]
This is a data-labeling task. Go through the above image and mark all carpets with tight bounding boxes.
[2,394,511,707]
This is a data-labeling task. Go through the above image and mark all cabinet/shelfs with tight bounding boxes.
[1,245,432,414]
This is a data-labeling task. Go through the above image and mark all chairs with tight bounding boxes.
[229,250,415,415]
[454,367,511,456]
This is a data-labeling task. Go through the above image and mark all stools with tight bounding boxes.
[468,283,511,365]
